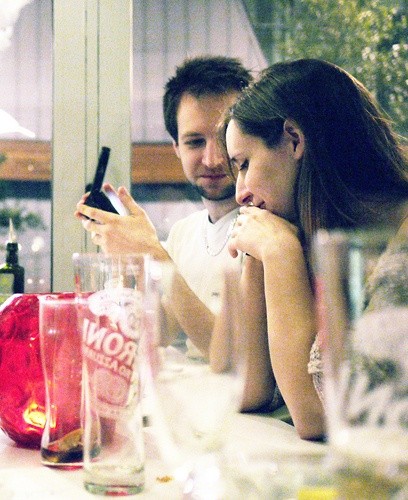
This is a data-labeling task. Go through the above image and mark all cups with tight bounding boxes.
[321,228,389,354]
[73,253,152,497]
[40,296,101,464]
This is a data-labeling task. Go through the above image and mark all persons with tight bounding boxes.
[215,57,408,441]
[73,53,270,378]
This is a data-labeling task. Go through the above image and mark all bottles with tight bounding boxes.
[0,242,25,306]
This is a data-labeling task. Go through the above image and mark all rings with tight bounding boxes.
[89,231,98,237]
[236,215,245,225]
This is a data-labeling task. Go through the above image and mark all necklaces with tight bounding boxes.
[192,200,242,258]
